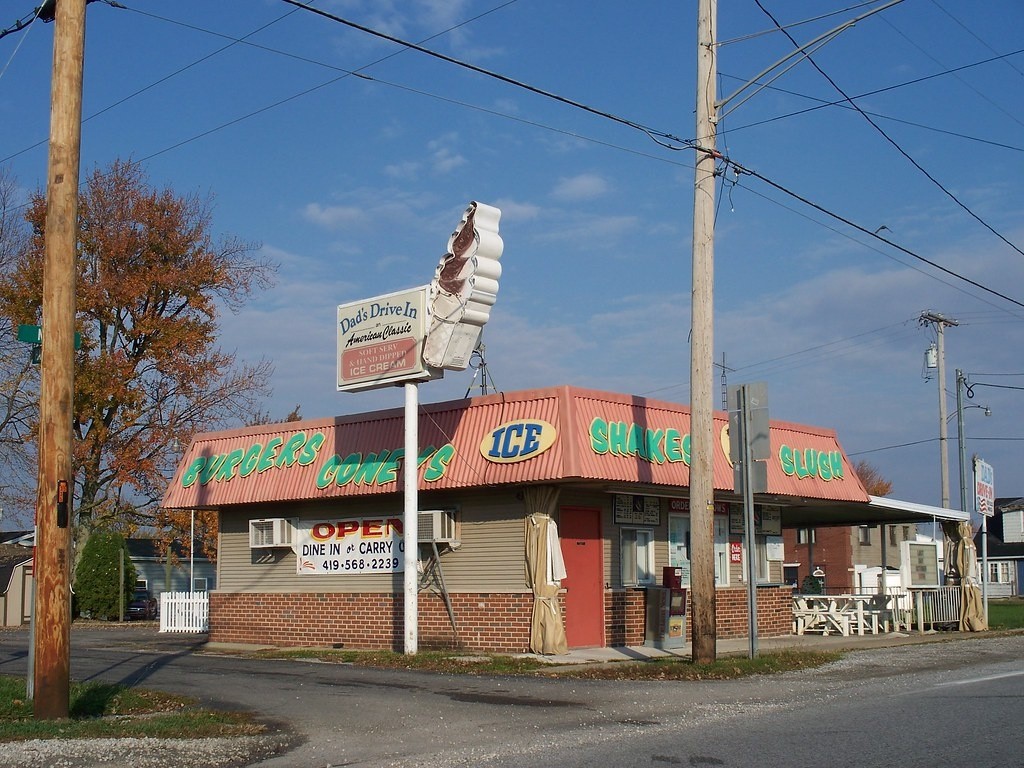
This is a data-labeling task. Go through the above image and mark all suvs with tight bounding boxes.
[108,586,159,621]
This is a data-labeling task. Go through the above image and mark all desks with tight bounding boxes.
[836,594,907,630]
[793,593,877,636]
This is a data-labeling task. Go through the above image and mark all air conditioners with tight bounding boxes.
[247,518,292,548]
[403,510,455,542]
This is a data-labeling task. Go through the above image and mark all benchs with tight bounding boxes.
[786,607,904,637]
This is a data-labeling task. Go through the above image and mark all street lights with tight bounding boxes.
[940,388,991,575]
